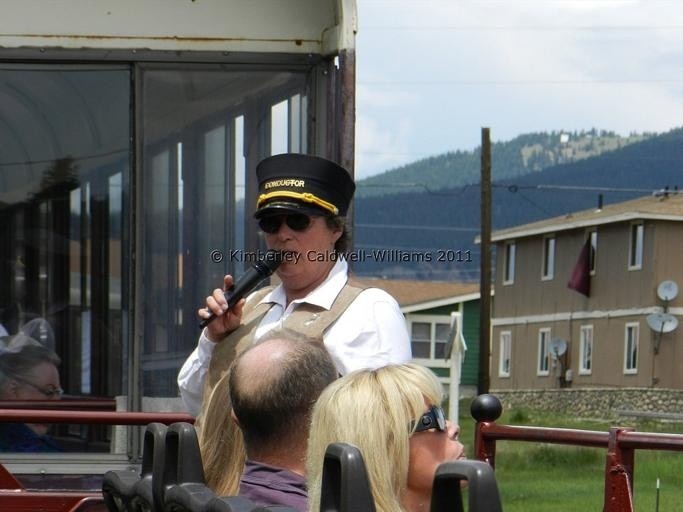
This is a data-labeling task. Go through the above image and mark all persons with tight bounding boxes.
[0,345,63,452]
[177,153,469,512]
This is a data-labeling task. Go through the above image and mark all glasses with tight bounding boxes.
[9,372,64,401]
[257,211,325,235]
[388,403,447,441]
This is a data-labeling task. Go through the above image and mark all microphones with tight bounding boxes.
[198,249,280,330]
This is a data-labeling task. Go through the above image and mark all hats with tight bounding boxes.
[254,154,357,222]
